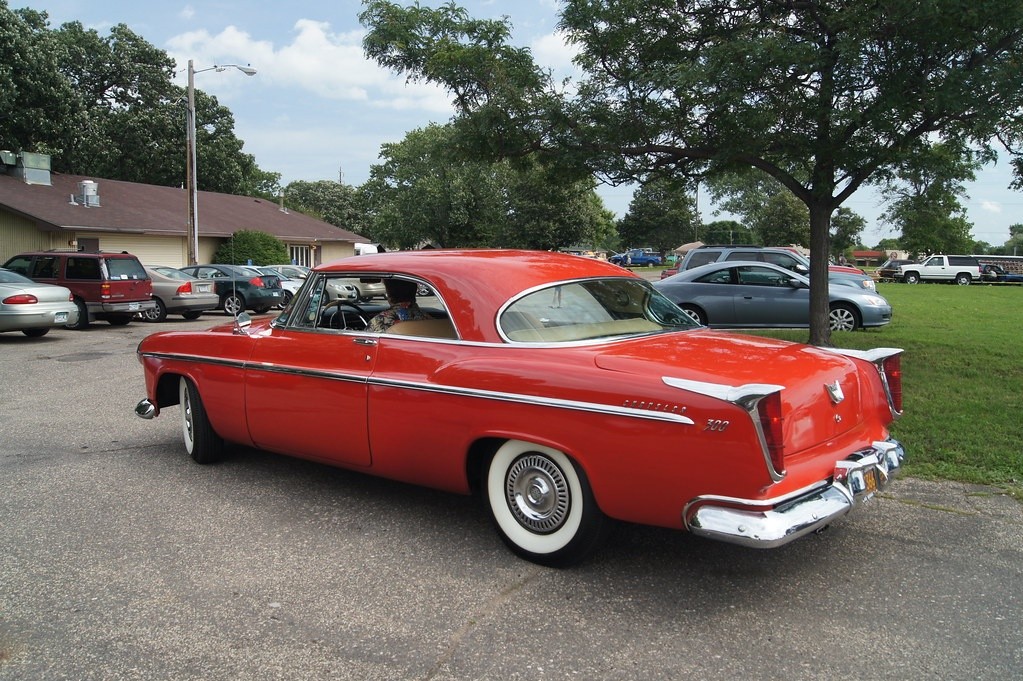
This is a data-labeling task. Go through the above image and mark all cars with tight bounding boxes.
[234,265,327,311]
[129,263,220,323]
[878,258,917,277]
[133,247,908,567]
[977,263,1023,283]
[262,264,358,306]
[0,268,79,339]
[647,259,893,333]
[677,248,878,296]
[783,248,869,276]
[176,263,286,318]
[663,250,682,267]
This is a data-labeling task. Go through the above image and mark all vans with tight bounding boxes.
[0,246,157,332]
[322,275,388,303]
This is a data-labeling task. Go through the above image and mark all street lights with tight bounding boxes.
[184,56,258,265]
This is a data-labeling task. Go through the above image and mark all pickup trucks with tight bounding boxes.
[608,249,663,268]
[894,254,984,287]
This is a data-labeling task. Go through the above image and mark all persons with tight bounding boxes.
[547,283,564,309]
[625,254,632,271]
[363,277,437,340]
